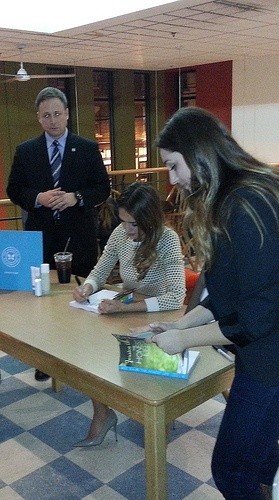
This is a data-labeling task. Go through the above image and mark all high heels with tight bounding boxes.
[72,409,119,448]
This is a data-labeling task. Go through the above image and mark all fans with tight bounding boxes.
[0,44,77,83]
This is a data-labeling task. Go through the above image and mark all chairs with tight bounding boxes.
[96,189,124,272]
[164,185,203,270]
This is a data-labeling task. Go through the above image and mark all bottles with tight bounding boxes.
[35,276,42,297]
[40,263,50,295]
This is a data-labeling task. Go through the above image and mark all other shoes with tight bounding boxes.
[35,369,51,381]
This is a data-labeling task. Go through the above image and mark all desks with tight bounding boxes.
[0,269,236,500]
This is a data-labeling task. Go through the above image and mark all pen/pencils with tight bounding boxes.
[212,344,234,362]
[74,274,91,304]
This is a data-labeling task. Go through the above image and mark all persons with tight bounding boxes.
[129,106,279,500]
[74,182,186,447]
[6,87,111,381]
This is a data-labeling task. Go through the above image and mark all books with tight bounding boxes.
[68,288,135,314]
[112,329,201,379]
[184,71,196,108]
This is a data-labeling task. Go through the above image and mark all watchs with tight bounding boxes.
[75,190,82,206]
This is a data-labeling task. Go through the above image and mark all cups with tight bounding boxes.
[54,252,73,283]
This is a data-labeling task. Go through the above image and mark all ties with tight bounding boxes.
[50,140,63,222]
